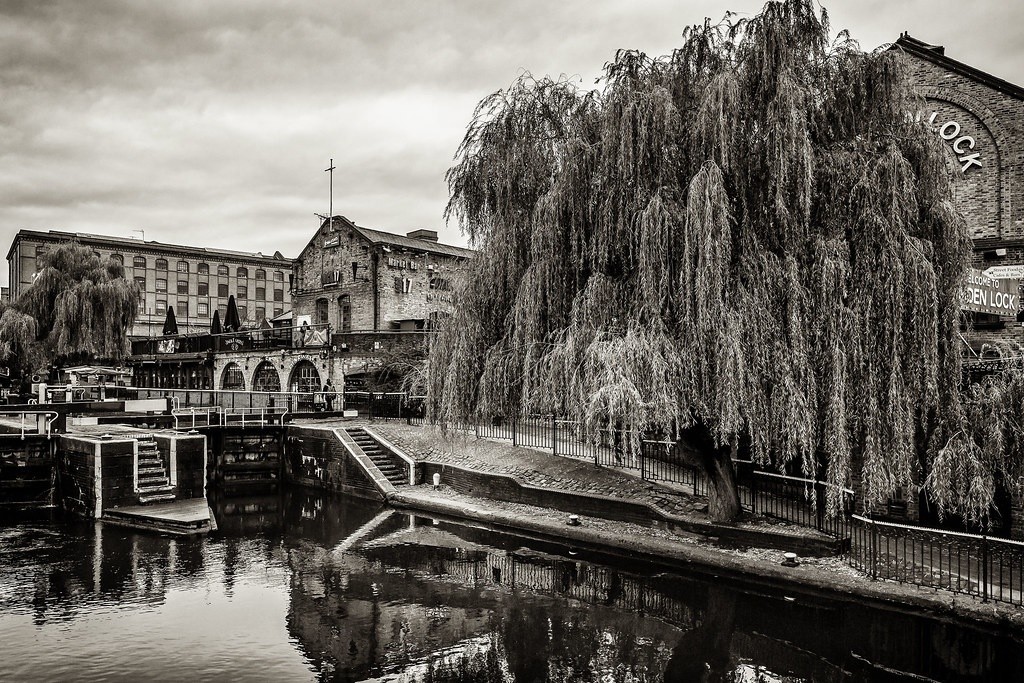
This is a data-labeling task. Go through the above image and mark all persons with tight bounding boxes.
[301,321,310,347]
[321,379,335,411]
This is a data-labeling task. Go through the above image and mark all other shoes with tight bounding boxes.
[330,409,334,411]
[325,409,329,411]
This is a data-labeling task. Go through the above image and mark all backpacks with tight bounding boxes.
[326,384,335,398]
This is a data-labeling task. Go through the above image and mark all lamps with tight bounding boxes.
[569,515,578,523]
[784,552,797,562]
